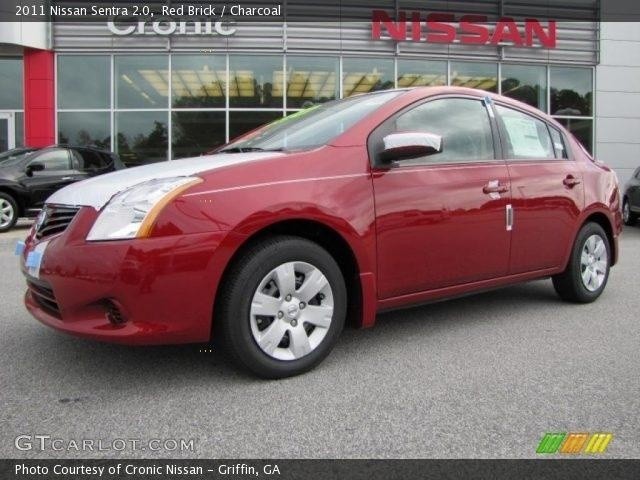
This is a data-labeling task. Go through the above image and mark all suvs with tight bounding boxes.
[0,143,127,236]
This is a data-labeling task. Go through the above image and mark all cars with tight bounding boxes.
[621,164,639,225]
[13,84,624,381]
